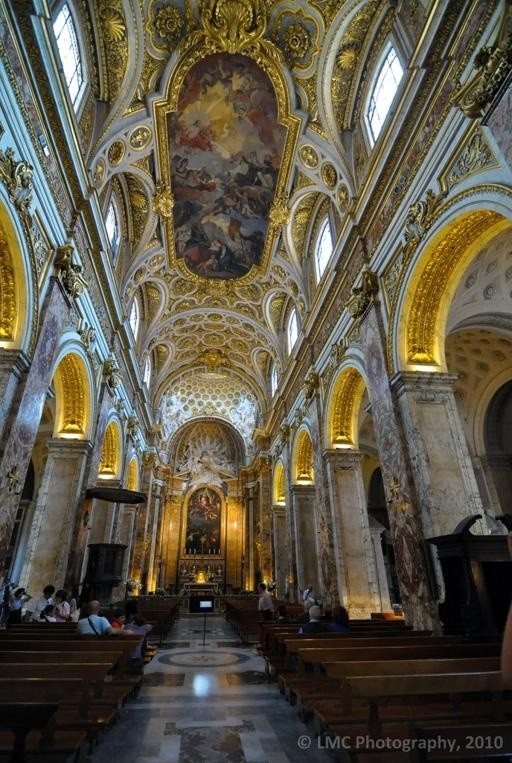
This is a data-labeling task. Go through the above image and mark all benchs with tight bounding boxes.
[225,600,512,763]
[0,600,178,763]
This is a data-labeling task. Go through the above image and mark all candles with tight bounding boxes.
[189,548,191,554]
[214,549,216,555]
[184,549,186,554]
[219,549,221,554]
[209,549,210,554]
[194,549,196,555]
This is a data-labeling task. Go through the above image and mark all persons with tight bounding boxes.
[0,567,152,676]
[252,582,276,656]
[167,52,287,275]
[186,488,221,554]
[273,582,351,669]
